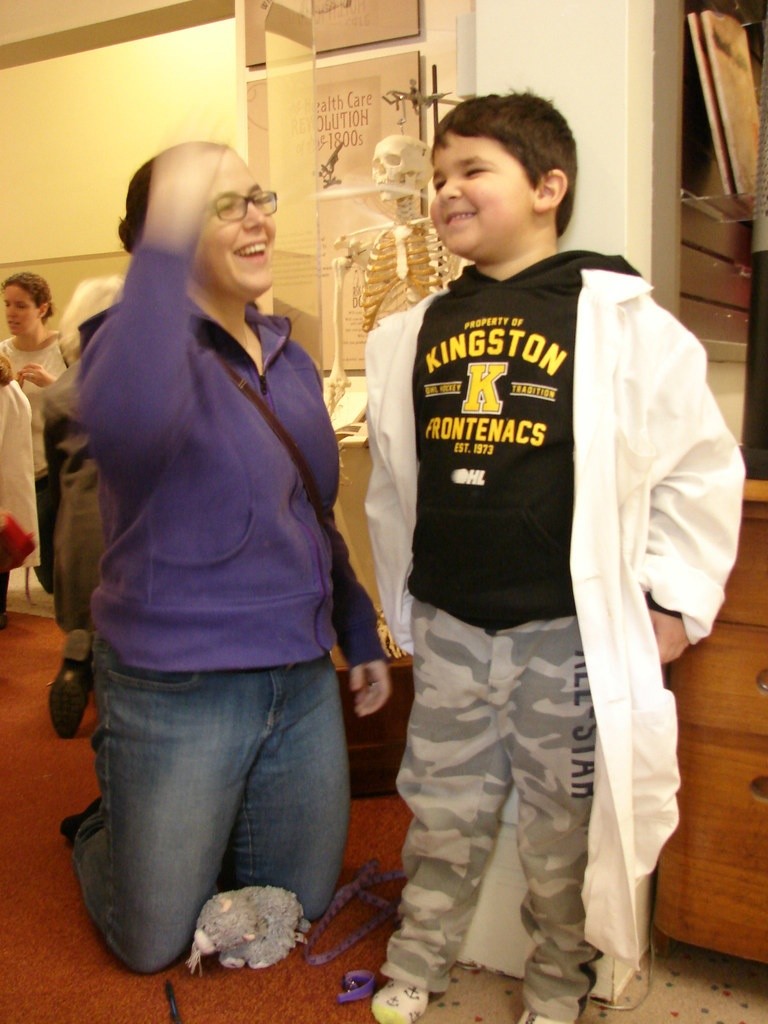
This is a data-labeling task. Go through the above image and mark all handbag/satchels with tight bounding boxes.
[0,513,36,573]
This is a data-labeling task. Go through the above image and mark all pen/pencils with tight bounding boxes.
[162,978,181,1024]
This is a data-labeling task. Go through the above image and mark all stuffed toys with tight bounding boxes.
[187,884,311,971]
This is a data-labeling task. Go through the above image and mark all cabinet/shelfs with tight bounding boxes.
[652,479,768,966]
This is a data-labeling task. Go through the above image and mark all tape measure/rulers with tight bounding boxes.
[303,857,406,966]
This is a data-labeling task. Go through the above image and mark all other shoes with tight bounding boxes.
[49,630,95,739]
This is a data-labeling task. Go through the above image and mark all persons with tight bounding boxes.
[62,141,389,974]
[365,92,744,1024]
[0,272,65,595]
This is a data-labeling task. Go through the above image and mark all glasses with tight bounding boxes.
[207,191,277,223]
